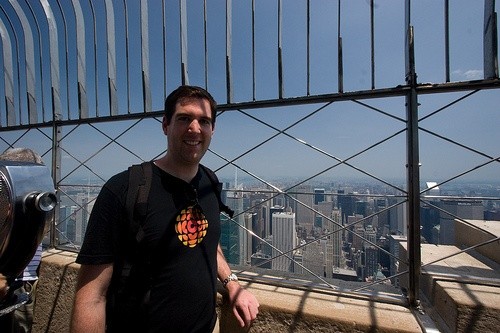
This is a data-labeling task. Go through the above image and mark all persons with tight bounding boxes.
[71,84,259,333]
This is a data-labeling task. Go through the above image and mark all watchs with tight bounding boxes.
[222,272,239,288]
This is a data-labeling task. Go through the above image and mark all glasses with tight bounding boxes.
[186,183,208,221]
